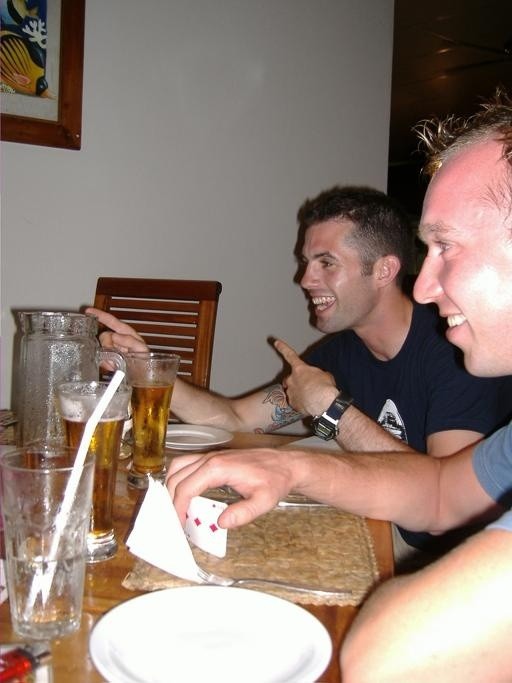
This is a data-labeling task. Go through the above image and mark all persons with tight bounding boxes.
[164,95,512,680]
[84,185,510,568]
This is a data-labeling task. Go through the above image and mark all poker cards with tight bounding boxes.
[184,496,229,559]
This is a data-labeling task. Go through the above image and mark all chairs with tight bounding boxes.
[92,276,221,417]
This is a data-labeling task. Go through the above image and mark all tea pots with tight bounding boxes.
[13,309,129,446]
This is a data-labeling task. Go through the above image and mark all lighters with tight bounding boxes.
[0,643,53,682]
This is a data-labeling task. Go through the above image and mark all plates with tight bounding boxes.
[87,585,334,683]
[164,423,235,452]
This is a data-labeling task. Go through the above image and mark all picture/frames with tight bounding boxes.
[0,0,86,151]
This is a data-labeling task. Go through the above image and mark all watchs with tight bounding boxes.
[312,390,356,442]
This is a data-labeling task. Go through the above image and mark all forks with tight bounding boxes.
[195,566,351,597]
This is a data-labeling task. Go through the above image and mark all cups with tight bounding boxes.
[56,381,133,564]
[126,352,183,490]
[0,446,97,640]
